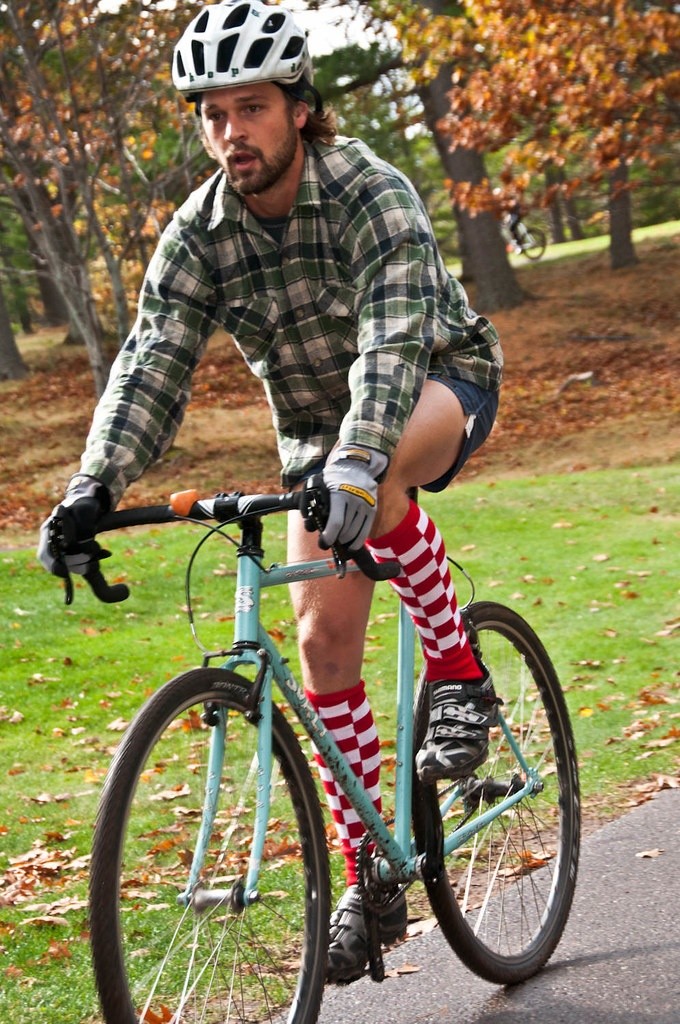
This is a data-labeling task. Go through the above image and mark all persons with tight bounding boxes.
[31,1,503,983]
[503,196,524,255]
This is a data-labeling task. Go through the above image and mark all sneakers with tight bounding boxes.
[319,884,407,984]
[416,657,504,781]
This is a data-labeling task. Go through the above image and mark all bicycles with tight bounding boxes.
[46,479,582,1024]
[496,196,547,261]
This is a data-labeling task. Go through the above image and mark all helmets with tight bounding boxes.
[173,0,313,101]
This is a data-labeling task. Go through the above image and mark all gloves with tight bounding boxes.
[37,473,113,576]
[318,443,389,555]
